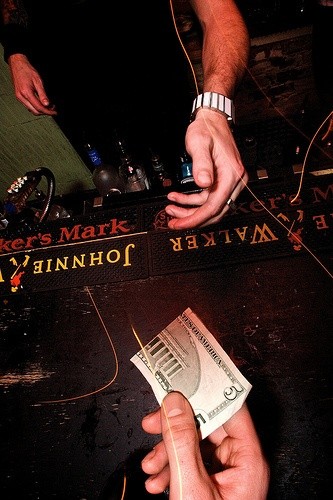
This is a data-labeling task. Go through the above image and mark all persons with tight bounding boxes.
[140,391,270,500]
[1,0,249,232]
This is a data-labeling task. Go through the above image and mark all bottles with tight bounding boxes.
[178,150,194,187]
[84,142,125,196]
[116,140,150,192]
[150,153,174,188]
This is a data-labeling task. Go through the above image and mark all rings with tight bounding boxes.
[227,199,237,213]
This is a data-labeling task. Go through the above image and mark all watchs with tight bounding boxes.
[191,91,239,131]
